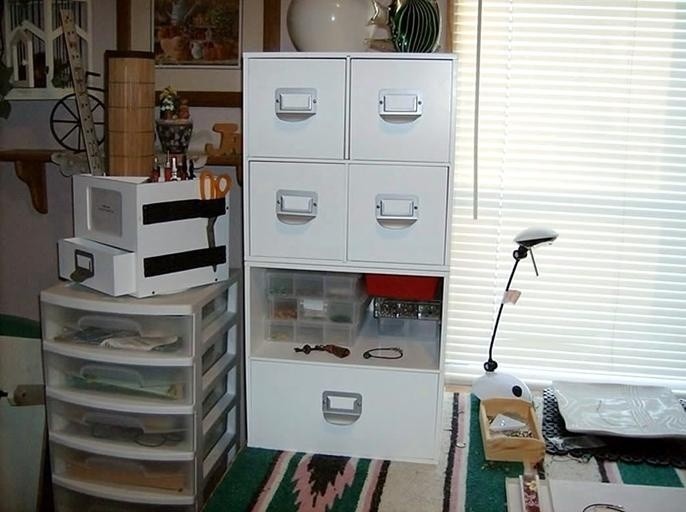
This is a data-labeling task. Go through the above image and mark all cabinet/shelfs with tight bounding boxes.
[39,266,242,512]
[240,49,456,469]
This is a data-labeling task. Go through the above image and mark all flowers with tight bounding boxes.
[159,83,189,119]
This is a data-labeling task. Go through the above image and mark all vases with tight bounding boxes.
[155,118,193,154]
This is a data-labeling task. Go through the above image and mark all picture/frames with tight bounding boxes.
[114,0,282,108]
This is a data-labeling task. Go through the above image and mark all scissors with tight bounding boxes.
[200,170,233,273]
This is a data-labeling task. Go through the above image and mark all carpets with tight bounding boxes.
[188,390,686,511]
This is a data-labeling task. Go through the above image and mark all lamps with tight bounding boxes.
[469,227,559,401]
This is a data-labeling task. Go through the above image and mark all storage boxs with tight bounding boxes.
[247,266,441,346]
